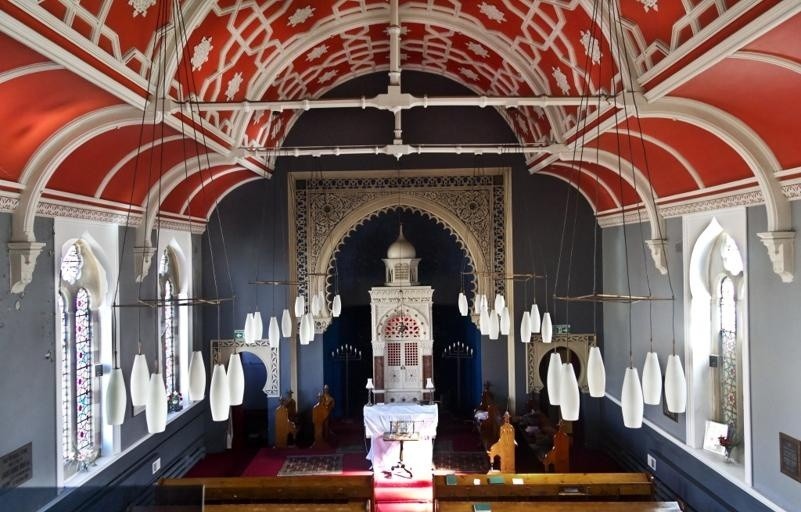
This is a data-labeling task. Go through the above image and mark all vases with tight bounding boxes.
[724,450,736,463]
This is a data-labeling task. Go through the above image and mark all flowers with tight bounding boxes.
[718,428,741,458]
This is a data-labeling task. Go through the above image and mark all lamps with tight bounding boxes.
[104,1,246,434]
[242,109,315,349]
[294,152,342,318]
[488,98,553,344]
[546,0,688,429]
[457,149,510,340]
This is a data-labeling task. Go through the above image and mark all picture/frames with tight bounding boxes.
[777,432,801,483]
